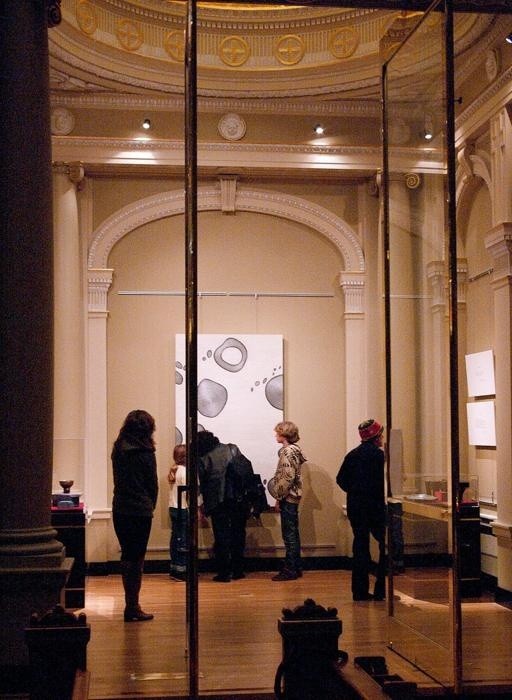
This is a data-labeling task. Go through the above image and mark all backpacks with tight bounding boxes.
[224,450,267,516]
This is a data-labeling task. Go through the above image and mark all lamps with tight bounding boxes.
[314,124,323,134]
[424,131,432,140]
[143,119,150,129]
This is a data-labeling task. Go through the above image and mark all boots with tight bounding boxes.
[124,575,153,621]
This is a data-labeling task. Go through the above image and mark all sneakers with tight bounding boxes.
[272,573,298,581]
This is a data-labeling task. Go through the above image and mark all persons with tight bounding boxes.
[112,407,159,623]
[334,418,390,602]
[270,421,308,581]
[165,443,191,584]
[197,429,251,582]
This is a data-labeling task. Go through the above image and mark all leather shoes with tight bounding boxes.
[232,574,245,579]
[213,575,231,582]
[354,593,374,602]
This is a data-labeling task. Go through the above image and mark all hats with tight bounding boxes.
[358,419,384,443]
